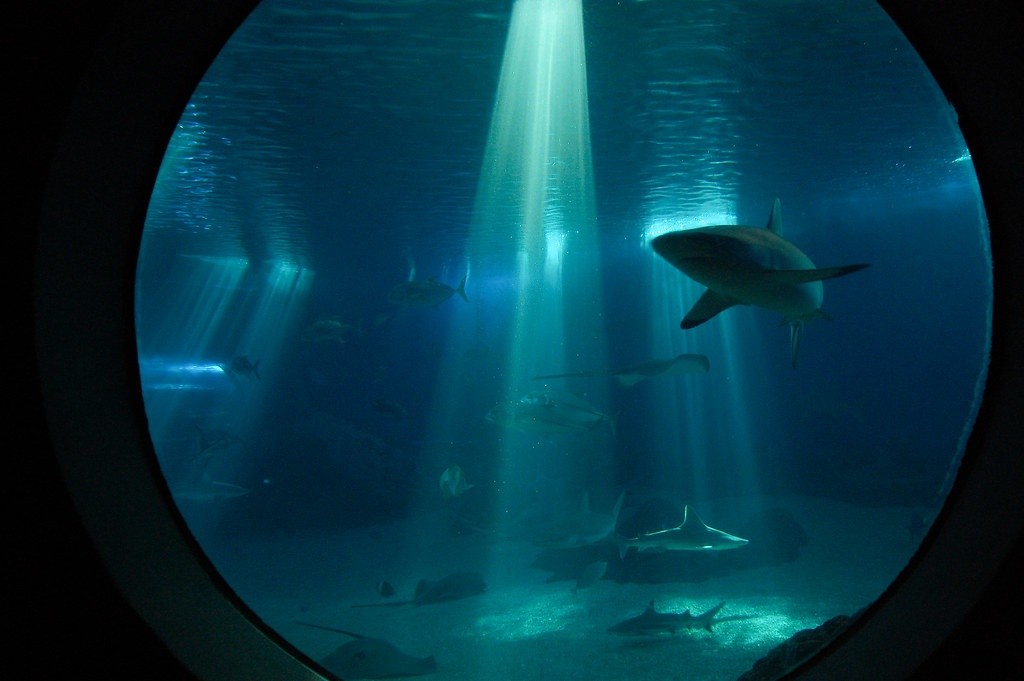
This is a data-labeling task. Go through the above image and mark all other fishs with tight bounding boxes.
[537,352,709,402]
[295,621,437,681]
[353,571,485,609]
